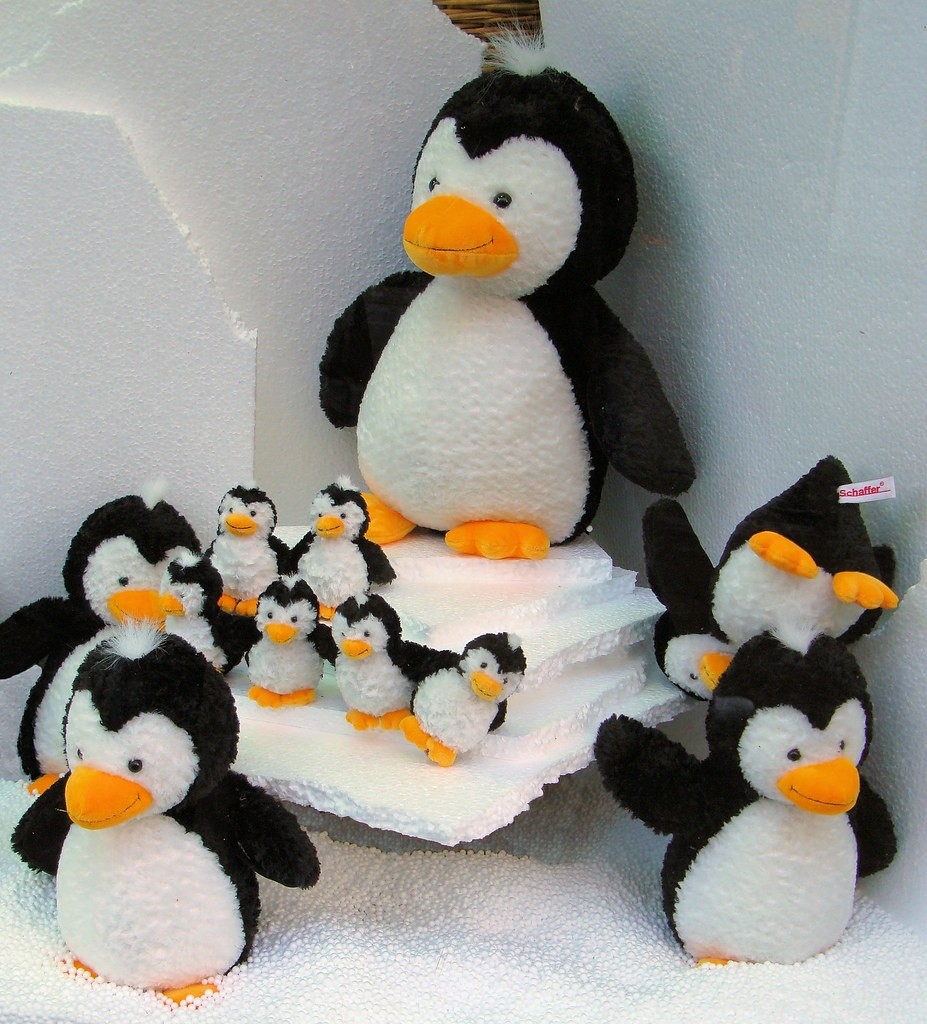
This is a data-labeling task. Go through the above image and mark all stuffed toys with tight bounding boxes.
[1,457,898,1011]
[319,18,697,560]
